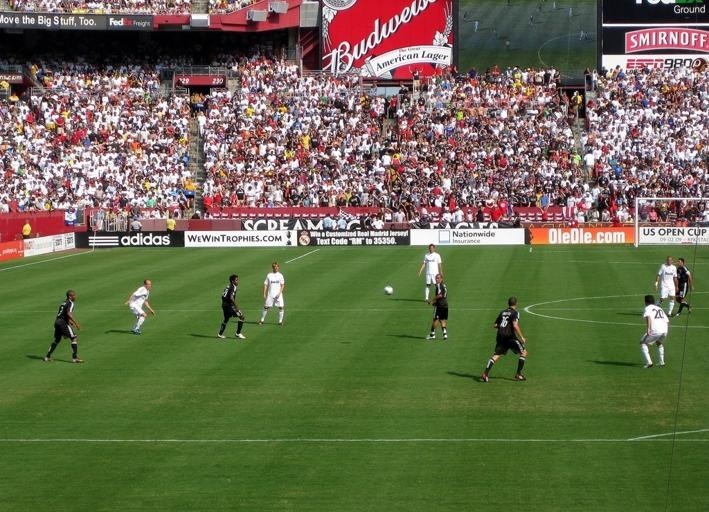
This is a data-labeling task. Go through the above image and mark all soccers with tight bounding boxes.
[384,285,394,295]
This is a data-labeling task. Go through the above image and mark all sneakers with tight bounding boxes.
[643,362,666,369]
[667,304,693,317]
[235,333,245,339]
[483,373,489,382]
[44,357,52,361]
[515,374,525,380]
[72,358,84,362]
[426,335,436,340]
[218,334,225,338]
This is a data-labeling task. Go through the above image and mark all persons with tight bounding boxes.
[124,279,156,336]
[43,290,84,363]
[426,274,449,340]
[418,244,444,305]
[2,1,263,15]
[375,60,709,229]
[217,274,246,339]
[2,34,395,229]
[640,295,669,368]
[655,256,679,317]
[256,262,285,326]
[479,297,528,382]
[674,258,693,317]
